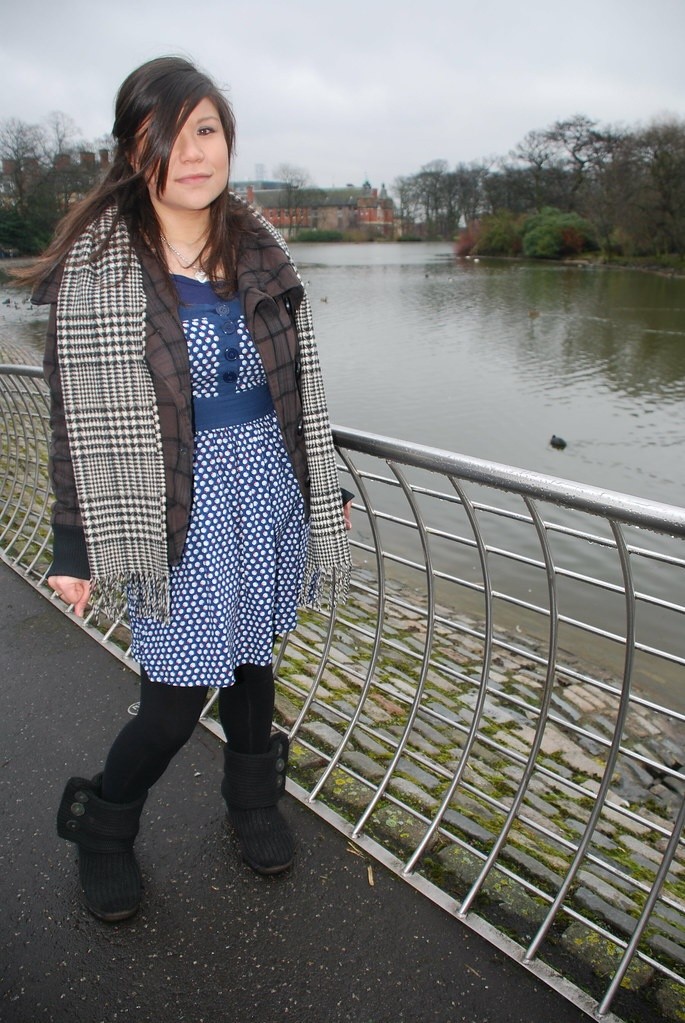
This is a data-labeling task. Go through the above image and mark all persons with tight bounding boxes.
[5,56,355,920]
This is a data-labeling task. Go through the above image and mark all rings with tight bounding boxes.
[54,591,62,597]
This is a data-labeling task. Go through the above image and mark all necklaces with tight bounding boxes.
[158,234,212,283]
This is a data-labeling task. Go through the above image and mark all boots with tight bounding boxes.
[220,733,294,874]
[56,772,148,922]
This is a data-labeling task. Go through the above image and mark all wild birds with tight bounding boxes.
[550,434,567,450]
